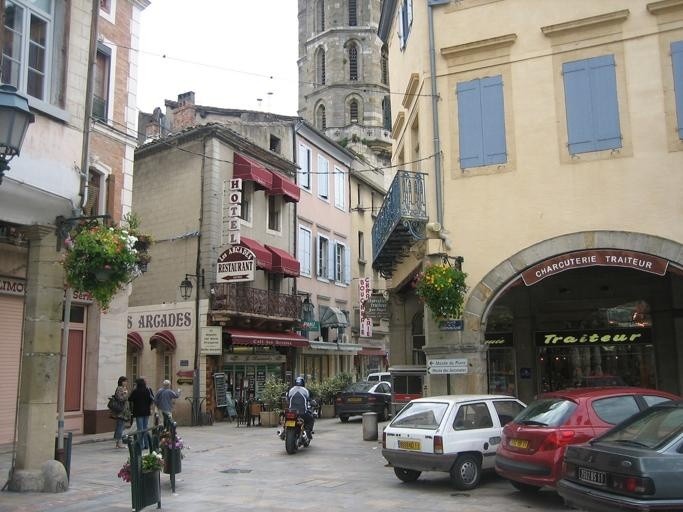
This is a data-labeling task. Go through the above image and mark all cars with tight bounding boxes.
[568,360,620,387]
[382,394,529,490]
[557,398,682,511]
[494,386,682,493]
[334,381,391,422]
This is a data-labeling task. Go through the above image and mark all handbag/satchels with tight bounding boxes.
[107,397,124,412]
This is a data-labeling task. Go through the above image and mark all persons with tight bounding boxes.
[154,379,181,425]
[127,377,154,448]
[108,377,131,449]
[278,377,315,439]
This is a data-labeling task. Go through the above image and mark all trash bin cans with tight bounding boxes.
[362,412,378,441]
[55,432,73,484]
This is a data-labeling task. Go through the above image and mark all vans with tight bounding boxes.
[365,371,391,384]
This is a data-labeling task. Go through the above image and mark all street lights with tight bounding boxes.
[0,84,36,189]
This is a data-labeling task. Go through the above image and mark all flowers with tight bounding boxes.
[156,431,185,451]
[118,452,164,483]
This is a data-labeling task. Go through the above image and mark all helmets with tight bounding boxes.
[295,377,305,386]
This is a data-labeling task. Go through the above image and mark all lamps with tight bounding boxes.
[298,293,315,318]
[178,273,205,300]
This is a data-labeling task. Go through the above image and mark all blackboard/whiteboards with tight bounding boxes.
[213,373,227,407]
[225,391,237,417]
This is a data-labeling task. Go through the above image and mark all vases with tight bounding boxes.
[163,448,182,492]
[131,470,161,512]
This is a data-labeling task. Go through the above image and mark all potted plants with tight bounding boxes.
[61,212,150,311]
[412,261,470,330]
[259,372,352,427]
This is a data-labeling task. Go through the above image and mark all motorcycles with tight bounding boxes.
[276,397,320,454]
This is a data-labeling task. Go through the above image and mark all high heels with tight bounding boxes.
[116,444,125,448]
